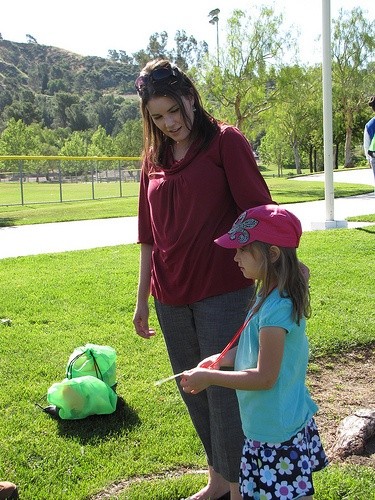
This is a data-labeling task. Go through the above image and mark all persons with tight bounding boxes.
[133,60,311,500]
[363,96,375,177]
[180,204,328,500]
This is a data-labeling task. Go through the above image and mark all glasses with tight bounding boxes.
[134,66,179,94]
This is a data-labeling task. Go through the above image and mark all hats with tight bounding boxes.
[214,204,302,248]
